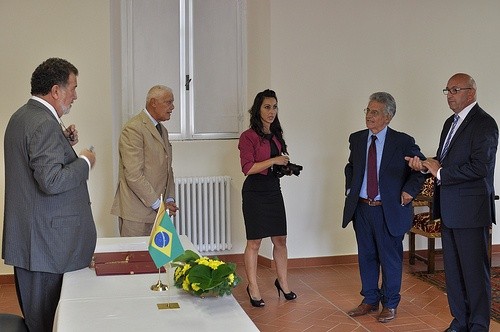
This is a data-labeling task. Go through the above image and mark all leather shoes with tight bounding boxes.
[377,306,397,323]
[347,302,379,316]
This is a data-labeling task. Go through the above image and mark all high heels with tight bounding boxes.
[246,285,265,306]
[274,279,296,300]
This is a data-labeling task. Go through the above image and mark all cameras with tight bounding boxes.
[276,161,303,176]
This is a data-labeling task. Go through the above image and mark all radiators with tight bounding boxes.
[166,175,234,253]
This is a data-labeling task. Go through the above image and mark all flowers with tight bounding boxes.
[171,250,241,296]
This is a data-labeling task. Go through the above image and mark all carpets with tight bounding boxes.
[411,266,500,324]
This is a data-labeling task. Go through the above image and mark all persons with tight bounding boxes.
[238,89,297,307]
[342,92,426,323]
[405,73,500,332]
[111,86,179,237]
[2,57,97,332]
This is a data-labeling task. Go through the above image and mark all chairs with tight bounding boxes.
[405,174,441,273]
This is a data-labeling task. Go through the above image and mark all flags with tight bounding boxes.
[148,209,184,269]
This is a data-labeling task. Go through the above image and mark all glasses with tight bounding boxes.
[443,87,471,95]
[364,109,385,116]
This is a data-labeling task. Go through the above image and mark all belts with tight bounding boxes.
[360,199,382,206]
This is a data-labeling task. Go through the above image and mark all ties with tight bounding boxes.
[440,115,459,163]
[156,122,163,137]
[367,135,378,200]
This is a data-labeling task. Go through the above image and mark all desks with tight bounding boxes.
[51,234,261,332]
